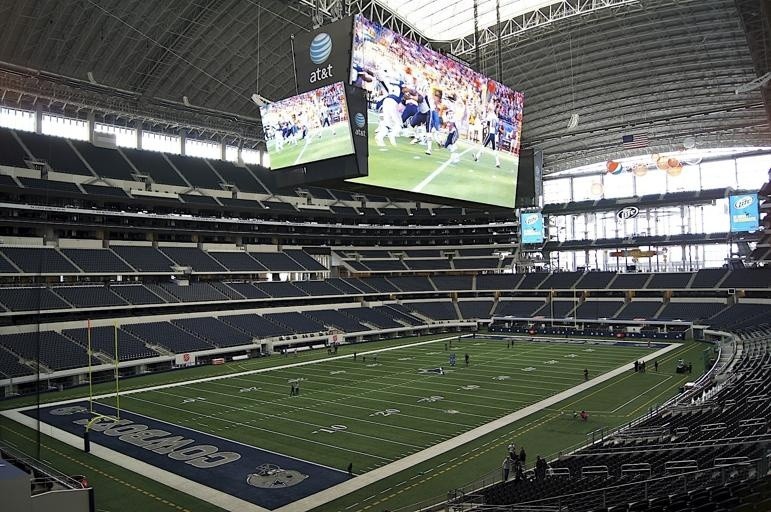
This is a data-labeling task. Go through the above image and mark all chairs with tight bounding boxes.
[427,346,769,509]
[2,130,538,408]
[541,265,771,346]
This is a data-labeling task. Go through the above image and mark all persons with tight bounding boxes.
[290,382,300,396]
[507,340,514,348]
[465,354,469,364]
[581,410,589,419]
[353,14,525,168]
[502,442,526,481]
[328,346,337,355]
[534,456,547,479]
[347,463,352,478]
[572,410,578,419]
[633,360,646,373]
[584,368,589,380]
[655,362,658,371]
[269,80,347,153]
